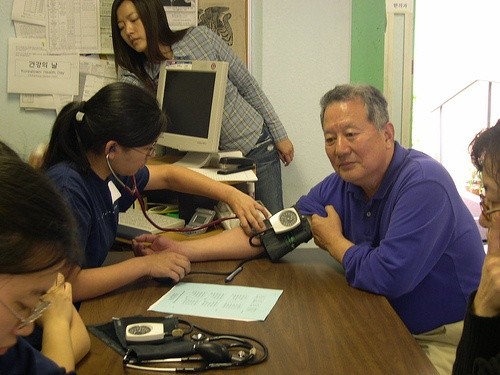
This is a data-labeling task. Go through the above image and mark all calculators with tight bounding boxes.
[167,207,216,237]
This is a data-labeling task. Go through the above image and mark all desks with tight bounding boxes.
[116,155,256,246]
[76,248,439,375]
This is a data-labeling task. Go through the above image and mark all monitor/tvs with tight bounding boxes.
[157,60,229,153]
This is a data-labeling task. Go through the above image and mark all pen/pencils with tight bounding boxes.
[224,266,244,283]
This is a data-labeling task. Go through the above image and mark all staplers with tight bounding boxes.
[217,156,256,174]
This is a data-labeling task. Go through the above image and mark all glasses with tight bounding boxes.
[132,145,156,159]
[479,193,500,221]
[0,298,51,330]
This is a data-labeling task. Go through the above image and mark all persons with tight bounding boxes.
[0,142,93,375]
[110,0,295,215]
[35,270,100,375]
[452,118,500,375]
[128,84,487,375]
[40,81,271,301]
